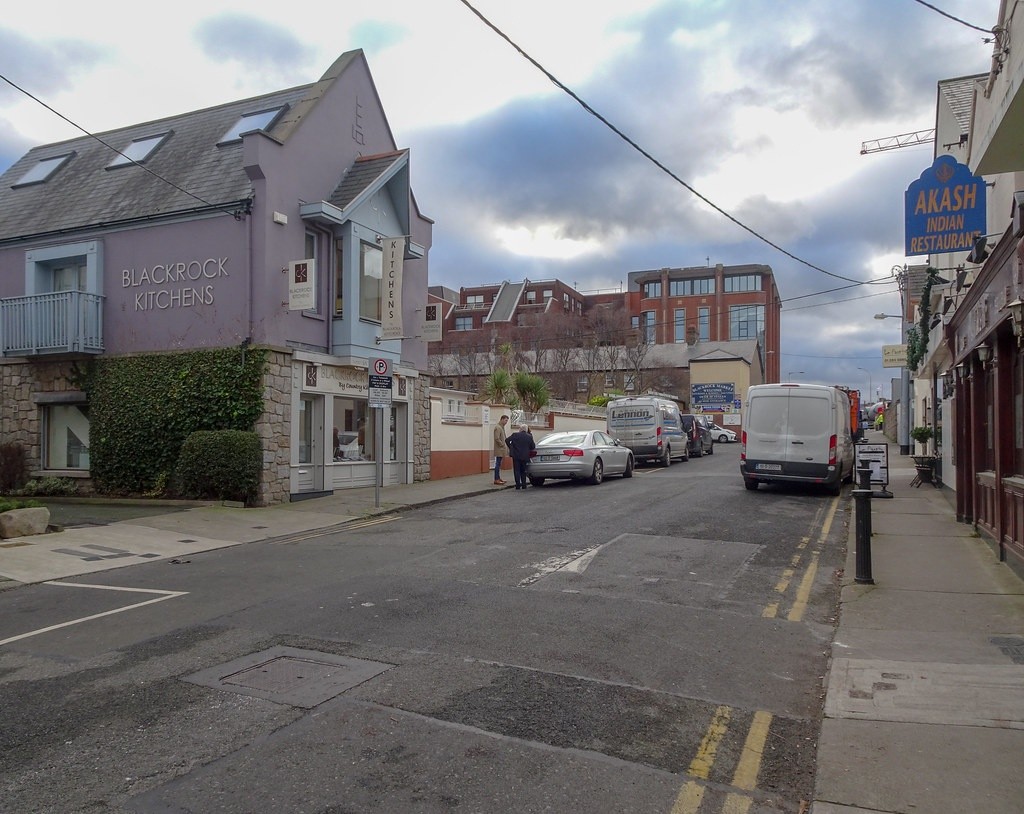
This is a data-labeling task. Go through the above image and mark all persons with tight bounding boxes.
[504,425,535,490]
[494,415,509,485]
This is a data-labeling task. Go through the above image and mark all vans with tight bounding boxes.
[606,396,692,469]
[681,413,713,457]
[740,383,856,496]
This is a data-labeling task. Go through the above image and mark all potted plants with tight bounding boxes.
[908,426,935,466]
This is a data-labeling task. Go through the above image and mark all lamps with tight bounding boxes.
[955,269,967,293]
[972,236,988,264]
[965,243,996,264]
[942,296,953,315]
[975,341,998,370]
[1005,295,1024,347]
[956,361,976,380]
[930,313,941,330]
[939,370,959,388]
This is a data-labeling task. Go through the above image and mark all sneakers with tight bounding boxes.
[516,485,520,490]
[522,485,527,489]
[494,479,507,485]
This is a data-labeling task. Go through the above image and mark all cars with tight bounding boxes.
[525,430,635,487]
[708,421,737,443]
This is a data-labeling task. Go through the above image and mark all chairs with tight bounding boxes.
[911,456,940,489]
